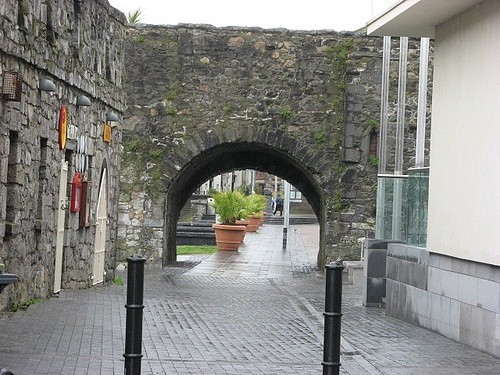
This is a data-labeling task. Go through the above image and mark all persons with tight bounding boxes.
[273,194,283,216]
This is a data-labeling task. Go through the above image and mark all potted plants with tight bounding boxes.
[211,191,250,250]
[246,193,266,232]
